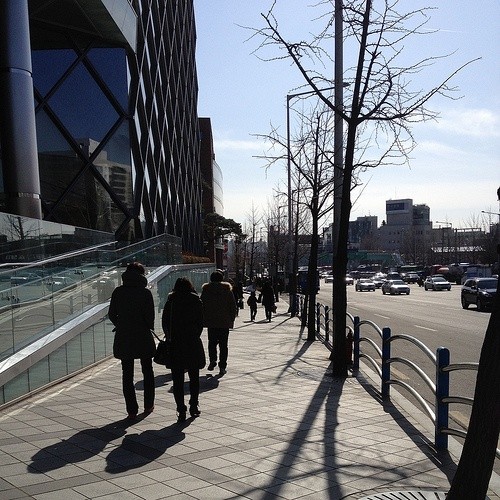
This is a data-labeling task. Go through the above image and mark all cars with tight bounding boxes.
[461,277,500,312]
[324,275,334,283]
[425,277,451,291]
[318,269,333,279]
[346,270,444,289]
[382,280,410,295]
[355,279,375,291]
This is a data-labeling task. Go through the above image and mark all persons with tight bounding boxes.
[248,280,280,322]
[201,267,243,375]
[108,262,156,420]
[162,275,205,421]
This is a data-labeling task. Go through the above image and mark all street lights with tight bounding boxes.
[292,187,313,239]
[276,204,297,302]
[267,214,287,286]
[436,220,452,264]
[287,82,351,312]
[481,211,500,216]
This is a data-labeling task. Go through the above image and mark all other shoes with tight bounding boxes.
[127,413,137,419]
[144,407,154,414]
[177,407,201,420]
[208,361,217,371]
[220,368,227,374]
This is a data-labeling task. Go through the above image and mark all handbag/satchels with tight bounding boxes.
[153,341,181,370]
